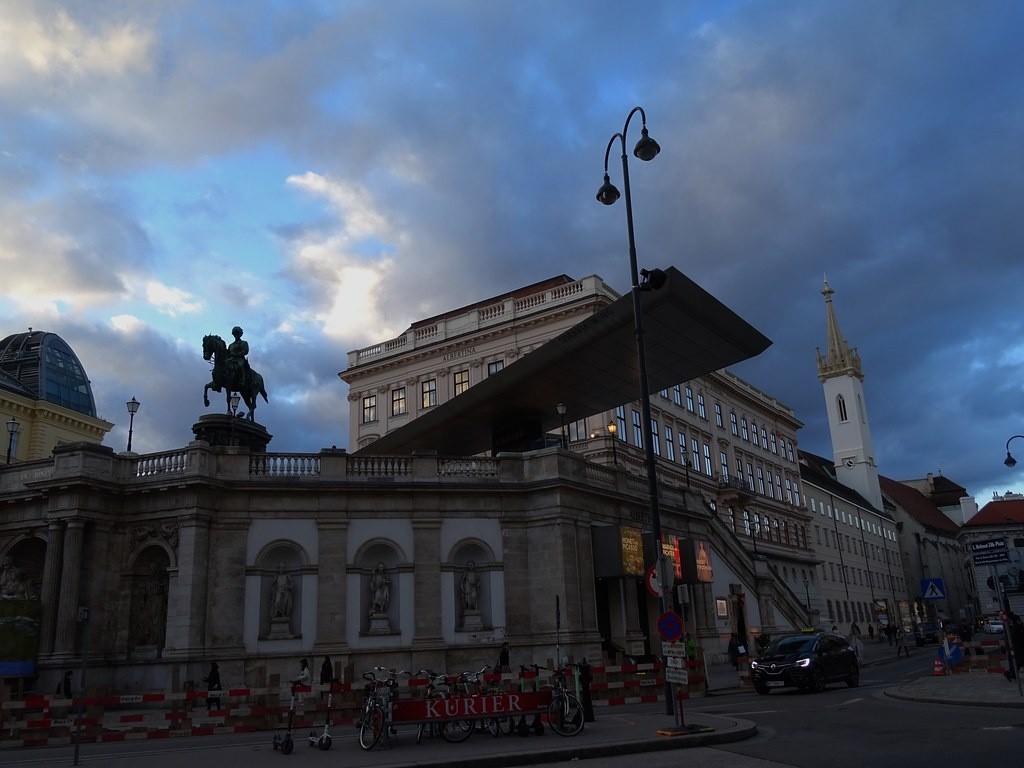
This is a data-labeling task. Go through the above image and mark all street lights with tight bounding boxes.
[6,416,21,465]
[125,395,141,451]
[607,419,618,465]
[749,520,758,555]
[595,105,676,716]
[555,400,569,449]
[230,391,241,446]
[802,576,811,609]
[681,447,691,488]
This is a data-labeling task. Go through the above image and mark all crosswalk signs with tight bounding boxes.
[920,577,947,600]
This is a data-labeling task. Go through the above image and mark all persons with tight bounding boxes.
[295,657,313,704]
[0,555,26,600]
[851,621,861,640]
[868,624,874,639]
[200,661,222,711]
[960,618,980,656]
[493,642,512,691]
[460,560,481,610]
[999,550,1024,593]
[140,562,167,646]
[832,626,841,636]
[728,633,743,672]
[228,327,249,389]
[271,562,294,618]
[884,623,911,658]
[369,563,392,616]
[687,633,696,670]
[1001,613,1024,682]
[56,671,74,699]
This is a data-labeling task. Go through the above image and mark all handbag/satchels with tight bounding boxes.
[493,664,501,674]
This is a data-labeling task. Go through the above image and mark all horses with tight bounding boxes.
[202,334,269,422]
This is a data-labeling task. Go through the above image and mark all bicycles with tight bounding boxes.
[373,663,586,748]
[356,672,396,751]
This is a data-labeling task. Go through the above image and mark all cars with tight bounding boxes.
[913,616,988,647]
[983,619,1005,634]
[750,625,859,694]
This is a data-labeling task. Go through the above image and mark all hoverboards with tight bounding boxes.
[273,679,305,755]
[309,676,344,751]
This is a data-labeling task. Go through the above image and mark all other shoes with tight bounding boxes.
[1004,671,1012,682]
